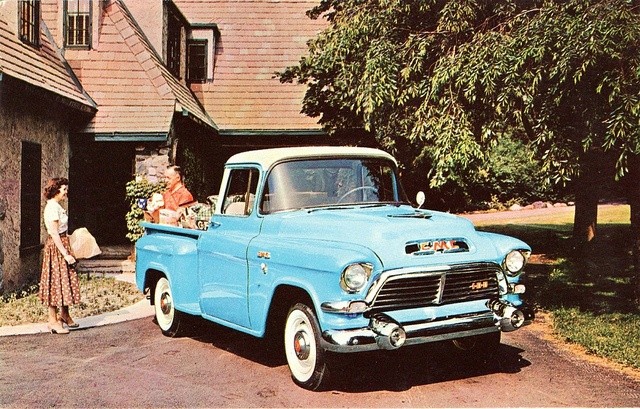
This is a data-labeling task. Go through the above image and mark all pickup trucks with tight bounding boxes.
[135,146,531,390]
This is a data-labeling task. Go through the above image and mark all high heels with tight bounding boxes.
[48,324,69,333]
[58,316,79,328]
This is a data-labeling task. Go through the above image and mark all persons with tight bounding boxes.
[137,165,194,227]
[39,177,81,334]
